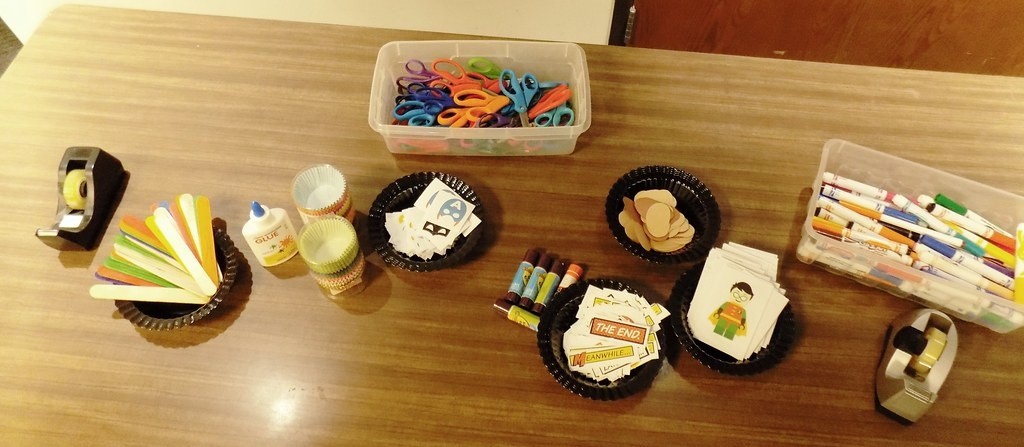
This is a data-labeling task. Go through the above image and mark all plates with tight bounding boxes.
[369,172,484,271]
[537,278,664,400]
[667,261,795,375]
[115,226,236,331]
[605,166,721,265]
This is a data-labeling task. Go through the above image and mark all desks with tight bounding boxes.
[0,0,1024,447]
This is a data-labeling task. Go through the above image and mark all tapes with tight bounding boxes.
[62,169,87,210]
[910,326,947,373]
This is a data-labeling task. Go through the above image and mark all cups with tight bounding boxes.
[289,163,356,223]
[296,215,366,292]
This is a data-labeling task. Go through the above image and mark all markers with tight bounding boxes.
[797,171,1024,330]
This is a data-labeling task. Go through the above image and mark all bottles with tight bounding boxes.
[241,201,299,267]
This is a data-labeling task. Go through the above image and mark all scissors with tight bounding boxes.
[391,59,574,152]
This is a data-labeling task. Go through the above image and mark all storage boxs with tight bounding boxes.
[368,40,591,156]
[796,139,1024,333]
